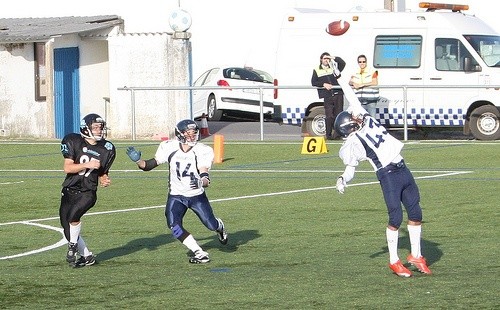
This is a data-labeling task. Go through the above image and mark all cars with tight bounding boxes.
[192,65,283,123]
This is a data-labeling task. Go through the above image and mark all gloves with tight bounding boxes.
[335,177,348,194]
[190,172,203,190]
[126,146,142,162]
[327,59,341,79]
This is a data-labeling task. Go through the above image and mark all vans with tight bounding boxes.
[274,1,500,141]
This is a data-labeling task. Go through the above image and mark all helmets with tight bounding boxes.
[175,119,199,146]
[333,111,359,138]
[79,113,105,140]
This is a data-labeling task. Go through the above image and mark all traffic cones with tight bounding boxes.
[199,113,210,136]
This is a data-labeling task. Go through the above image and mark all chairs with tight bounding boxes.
[436,45,457,70]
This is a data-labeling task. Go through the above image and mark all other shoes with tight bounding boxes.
[327,135,341,140]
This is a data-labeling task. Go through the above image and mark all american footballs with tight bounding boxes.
[325,20,350,36]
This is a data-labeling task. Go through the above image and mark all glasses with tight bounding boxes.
[358,61,366,64]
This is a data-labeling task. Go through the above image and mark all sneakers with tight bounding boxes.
[389,259,413,278]
[66,242,78,263]
[216,218,228,245]
[188,251,209,263]
[74,252,95,266]
[406,253,432,274]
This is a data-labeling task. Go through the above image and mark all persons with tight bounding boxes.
[327,59,434,278]
[58,113,117,267]
[310,52,346,141]
[125,119,228,263]
[348,54,380,121]
